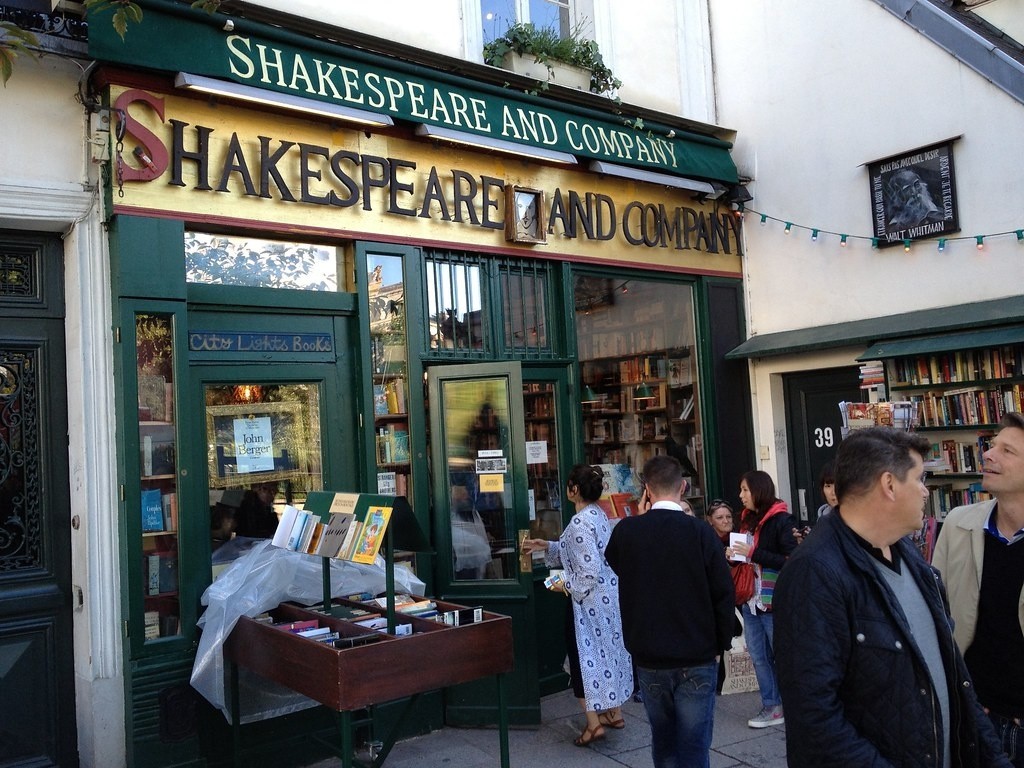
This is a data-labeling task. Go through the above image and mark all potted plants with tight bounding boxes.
[484,17,615,91]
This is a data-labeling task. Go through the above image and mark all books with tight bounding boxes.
[130,378,186,642]
[371,342,703,583]
[254,590,486,651]
[270,502,393,566]
[837,343,1024,565]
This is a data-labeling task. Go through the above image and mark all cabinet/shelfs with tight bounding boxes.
[521,381,560,511]
[371,373,411,501]
[226,491,516,768]
[133,311,180,642]
[579,344,707,519]
[881,354,1024,530]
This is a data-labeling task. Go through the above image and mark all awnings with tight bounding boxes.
[720,292,1024,363]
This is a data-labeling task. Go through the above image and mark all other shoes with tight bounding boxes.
[634,690,643,703]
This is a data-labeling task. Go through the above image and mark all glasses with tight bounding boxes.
[707,500,731,516]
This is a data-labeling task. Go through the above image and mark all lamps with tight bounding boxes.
[720,185,753,203]
[415,122,578,165]
[589,161,715,195]
[694,183,727,204]
[174,72,394,130]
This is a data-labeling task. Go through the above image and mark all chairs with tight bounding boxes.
[907,517,935,565]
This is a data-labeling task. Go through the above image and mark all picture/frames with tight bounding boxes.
[206,401,310,488]
[505,185,547,245]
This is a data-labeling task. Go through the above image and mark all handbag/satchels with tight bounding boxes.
[449,509,493,572]
[731,563,758,606]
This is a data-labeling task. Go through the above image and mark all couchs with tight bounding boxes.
[718,647,761,695]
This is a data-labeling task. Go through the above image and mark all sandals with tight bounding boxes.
[597,713,625,729]
[573,725,606,747]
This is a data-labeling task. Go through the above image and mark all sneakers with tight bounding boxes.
[747,704,784,728]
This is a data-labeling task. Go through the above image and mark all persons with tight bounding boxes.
[882,168,944,235]
[453,438,508,582]
[928,412,1023,768]
[519,468,625,749]
[764,431,1011,768]
[204,475,281,550]
[595,441,839,768]
